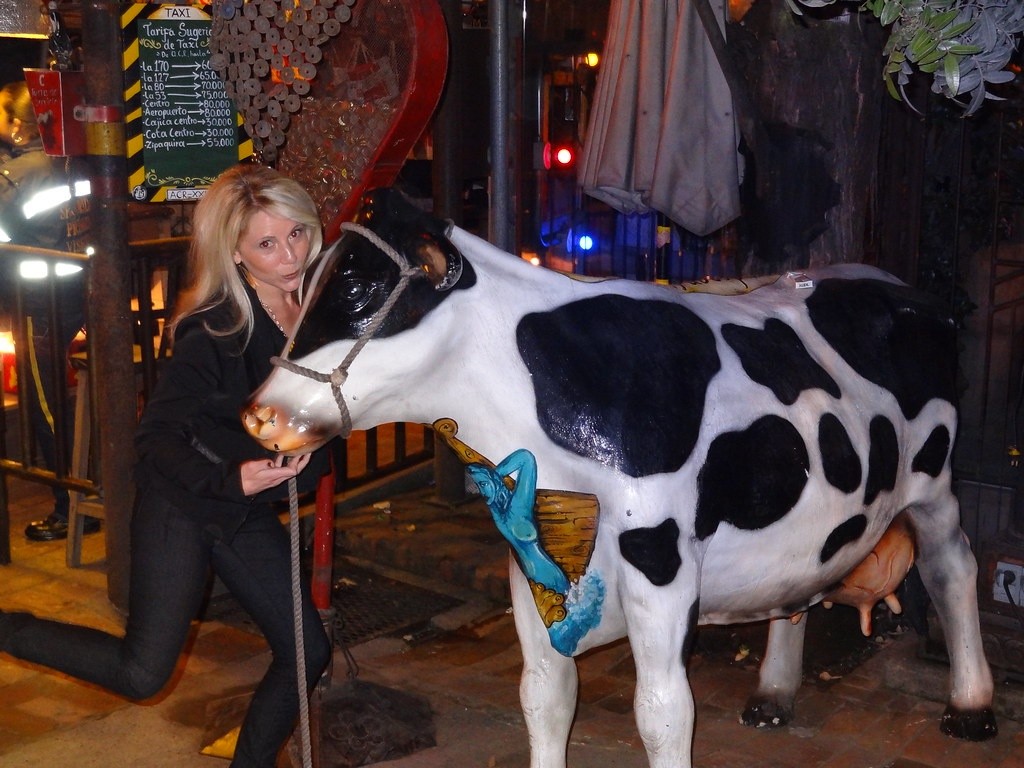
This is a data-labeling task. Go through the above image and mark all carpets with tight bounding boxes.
[2,647,653,767]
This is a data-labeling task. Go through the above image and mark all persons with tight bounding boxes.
[1,160,340,768]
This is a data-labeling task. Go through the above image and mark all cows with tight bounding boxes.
[237,156,1003,768]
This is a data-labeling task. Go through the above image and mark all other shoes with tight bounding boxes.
[27,509,101,539]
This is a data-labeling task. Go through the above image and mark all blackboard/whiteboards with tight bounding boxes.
[119,1,260,206]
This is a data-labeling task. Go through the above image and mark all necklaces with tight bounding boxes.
[258,298,289,341]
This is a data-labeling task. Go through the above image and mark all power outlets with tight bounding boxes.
[994,561,1020,606]
